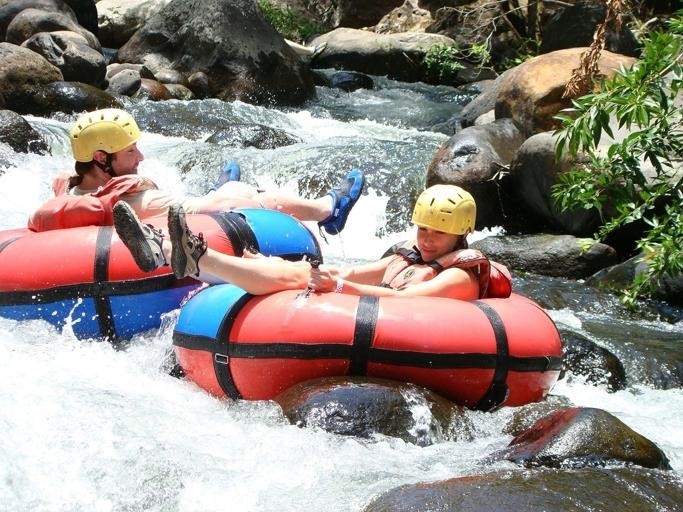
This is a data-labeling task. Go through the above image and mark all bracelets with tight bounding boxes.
[334,277,345,294]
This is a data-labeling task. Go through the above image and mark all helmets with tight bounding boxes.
[70,108,140,163]
[410,184,477,237]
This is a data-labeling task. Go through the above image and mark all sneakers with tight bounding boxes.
[318,168,364,234]
[168,202,208,278]
[205,162,240,197]
[112,199,169,273]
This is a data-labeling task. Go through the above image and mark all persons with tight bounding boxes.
[112,183,489,301]
[49,108,365,237]
